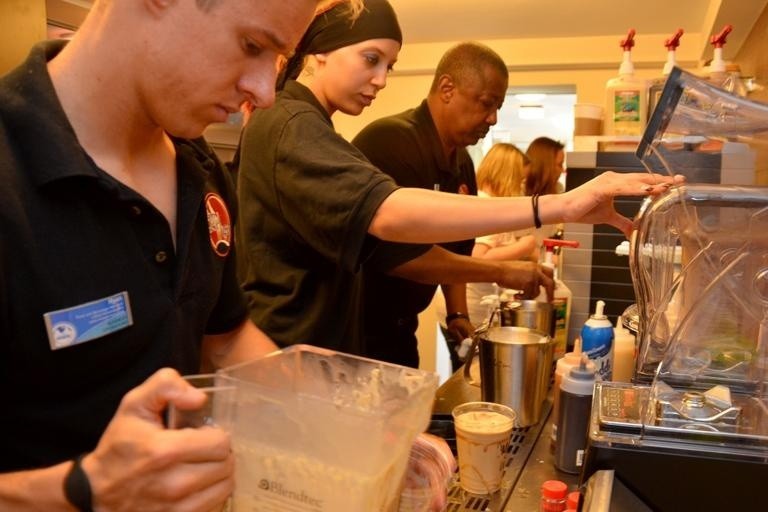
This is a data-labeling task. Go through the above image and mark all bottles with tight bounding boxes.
[538,479,568,512]
[604,23,750,147]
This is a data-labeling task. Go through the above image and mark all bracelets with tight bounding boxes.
[527,189,545,232]
[444,311,469,327]
[62,446,97,512]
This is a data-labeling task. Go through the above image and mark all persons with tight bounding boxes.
[445,140,544,380]
[521,136,569,194]
[350,41,510,366]
[232,0,689,359]
[0,1,461,512]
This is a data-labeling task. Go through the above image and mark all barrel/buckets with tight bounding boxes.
[463,324,553,430]
[488,301,557,341]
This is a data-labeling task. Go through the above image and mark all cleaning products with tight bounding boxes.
[699,24,732,88]
[536,238,581,361]
[646,27,682,123]
[602,29,648,141]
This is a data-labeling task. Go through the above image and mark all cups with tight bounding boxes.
[167,340,441,512]
[452,403,517,496]
[399,432,453,512]
[571,101,602,136]
